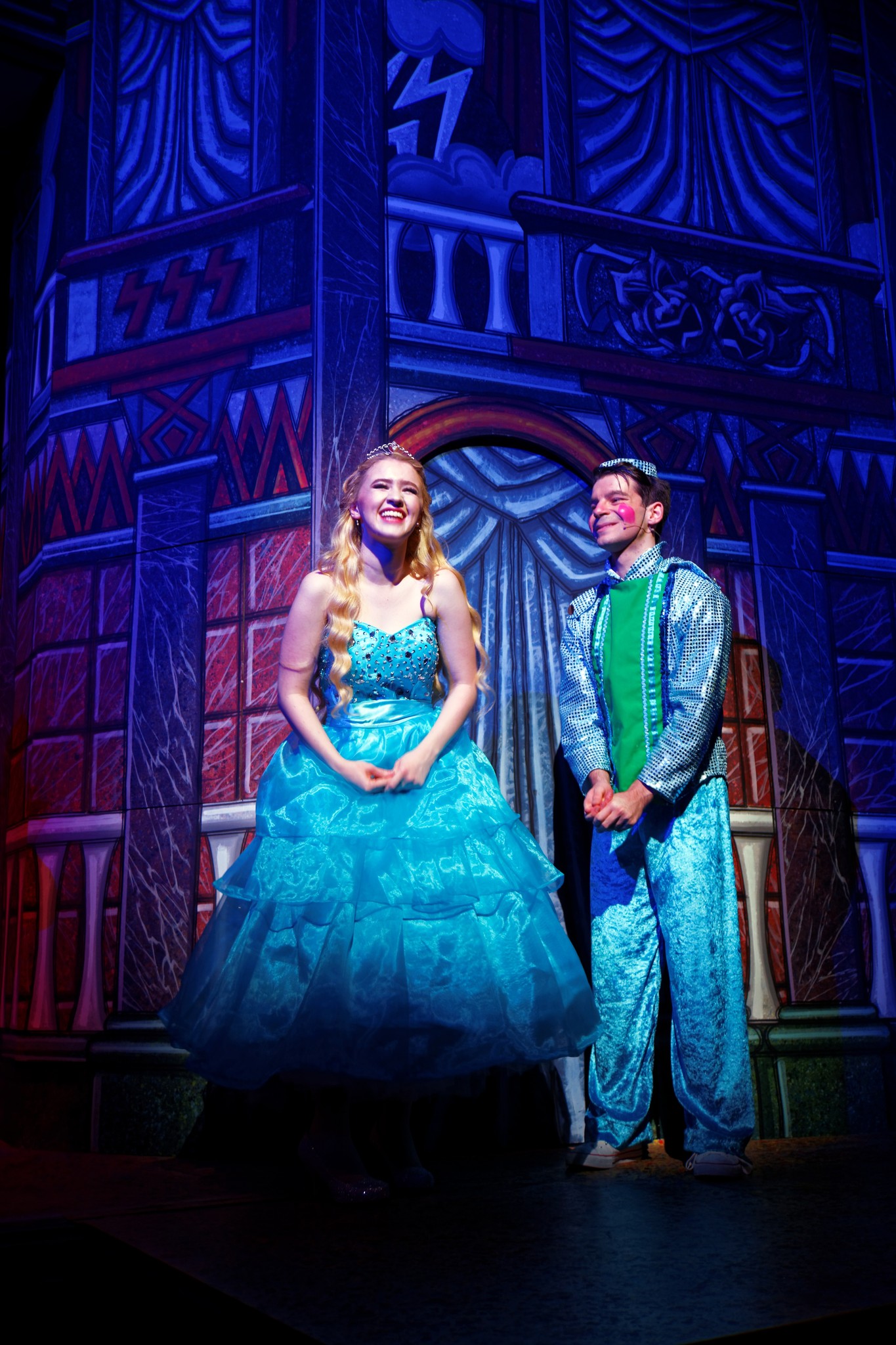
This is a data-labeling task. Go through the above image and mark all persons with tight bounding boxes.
[154,444,601,1088]
[557,460,757,1170]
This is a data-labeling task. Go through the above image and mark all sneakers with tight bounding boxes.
[585,1138,644,1166]
[690,1152,741,1177]
